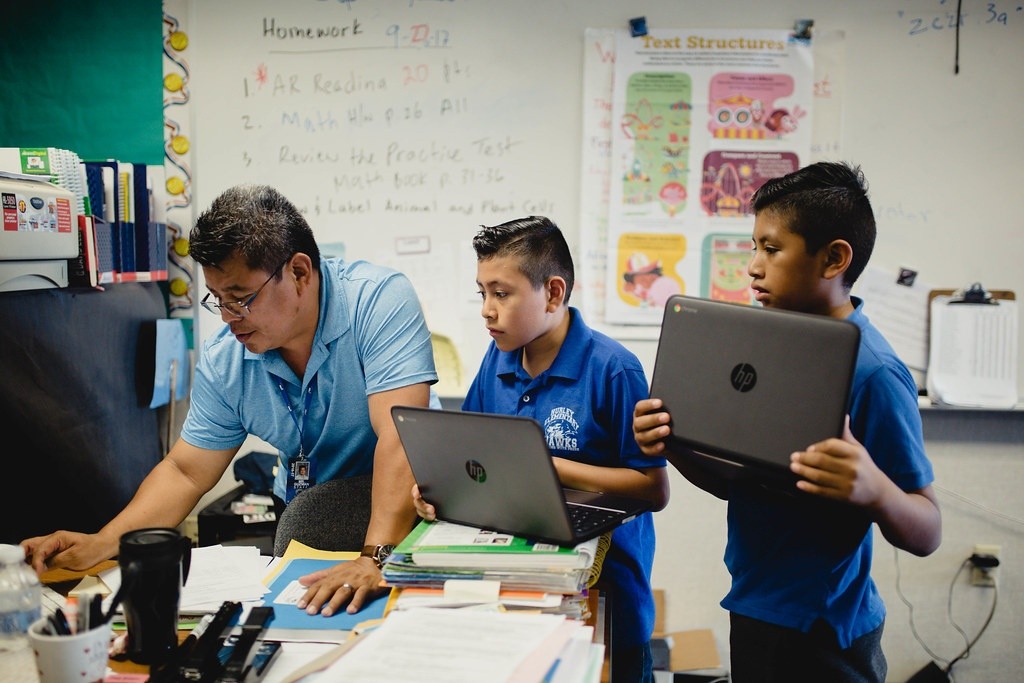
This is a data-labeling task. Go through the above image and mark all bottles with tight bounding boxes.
[0,544,42,683]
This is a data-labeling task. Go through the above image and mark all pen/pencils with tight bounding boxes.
[26,588,118,638]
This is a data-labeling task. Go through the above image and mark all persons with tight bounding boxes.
[19,183,438,617]
[412,215,671,682]
[632,159,944,683]
[296,468,308,481]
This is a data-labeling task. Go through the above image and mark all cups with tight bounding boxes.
[28,619,111,683]
[118,528,193,665]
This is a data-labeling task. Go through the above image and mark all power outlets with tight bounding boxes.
[969,543,1001,587]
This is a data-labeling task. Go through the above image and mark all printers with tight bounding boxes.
[0,178,79,292]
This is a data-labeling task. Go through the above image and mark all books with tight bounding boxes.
[305,512,606,683]
[0,147,168,285]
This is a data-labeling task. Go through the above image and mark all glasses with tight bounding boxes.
[200,258,285,318]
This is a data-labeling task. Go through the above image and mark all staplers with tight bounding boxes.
[180,600,283,683]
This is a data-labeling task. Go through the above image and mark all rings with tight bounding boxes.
[341,583,356,593]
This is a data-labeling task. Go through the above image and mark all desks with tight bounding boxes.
[0,561,600,683]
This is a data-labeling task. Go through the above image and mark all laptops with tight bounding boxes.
[649,294,861,514]
[390,405,651,548]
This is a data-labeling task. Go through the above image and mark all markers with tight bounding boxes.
[168,612,216,662]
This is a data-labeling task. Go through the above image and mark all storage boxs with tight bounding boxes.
[651,588,723,683]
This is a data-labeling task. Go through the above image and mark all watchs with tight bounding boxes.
[363,541,397,570]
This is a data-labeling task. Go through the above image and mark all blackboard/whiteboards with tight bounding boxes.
[162,0,1024,428]
